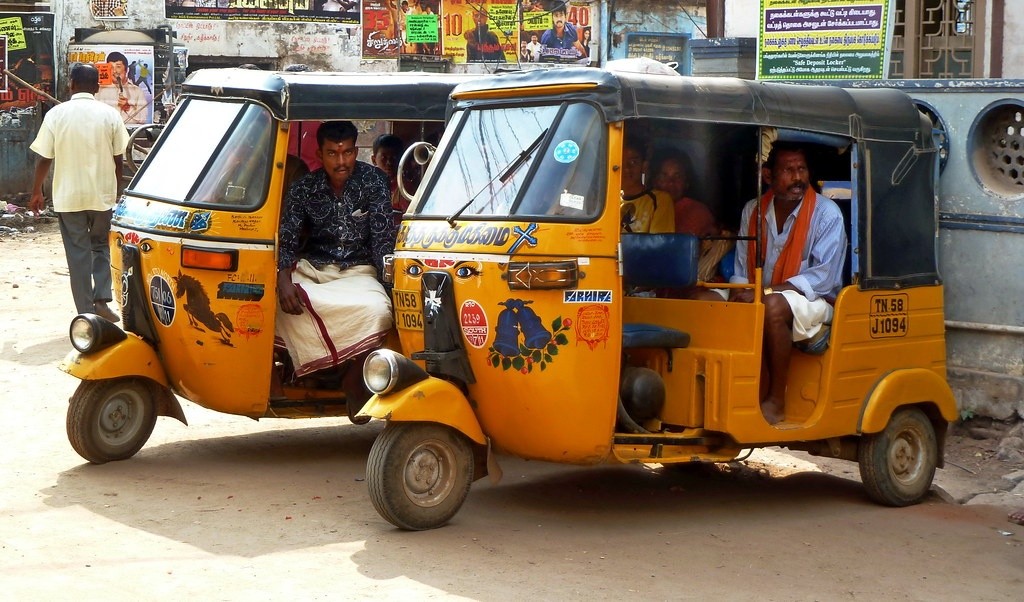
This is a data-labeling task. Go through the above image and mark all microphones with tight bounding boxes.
[116,77,123,97]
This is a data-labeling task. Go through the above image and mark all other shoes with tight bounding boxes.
[760,400,785,424]
[94,304,120,323]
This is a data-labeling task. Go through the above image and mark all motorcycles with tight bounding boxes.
[55,60,495,464]
[352,65,960,532]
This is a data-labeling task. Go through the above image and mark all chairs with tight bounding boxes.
[621,232,698,350]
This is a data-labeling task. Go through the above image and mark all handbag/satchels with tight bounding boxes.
[698,230,735,281]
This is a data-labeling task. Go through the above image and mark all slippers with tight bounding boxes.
[343,380,372,424]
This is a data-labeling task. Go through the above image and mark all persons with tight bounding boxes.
[726,144,845,423]
[463,6,507,63]
[646,148,720,246]
[388,0,438,55]
[521,1,591,63]
[275,121,396,426]
[96,51,148,123]
[27,64,129,325]
[206,111,310,221]
[609,140,675,234]
[370,132,414,212]
